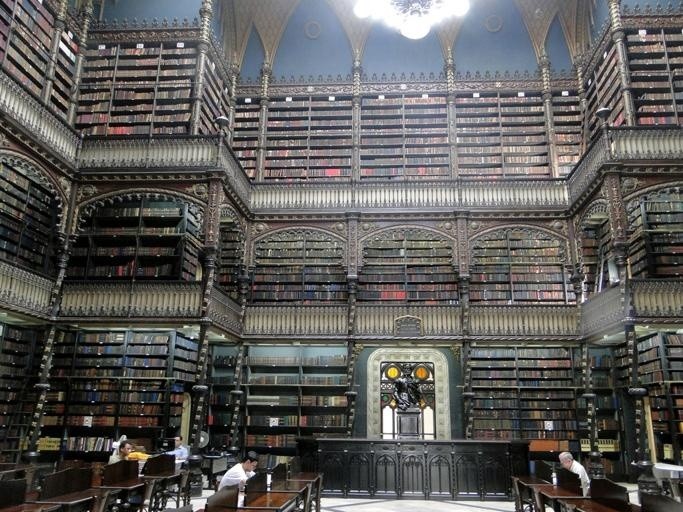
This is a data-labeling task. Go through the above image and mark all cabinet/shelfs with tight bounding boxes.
[0,0,681,464]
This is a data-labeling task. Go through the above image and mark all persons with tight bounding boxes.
[217,453,257,493]
[559,451,591,497]
[108,441,142,509]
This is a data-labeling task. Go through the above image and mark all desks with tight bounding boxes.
[238,471,325,511]
[510,473,617,510]
[2,472,188,511]
